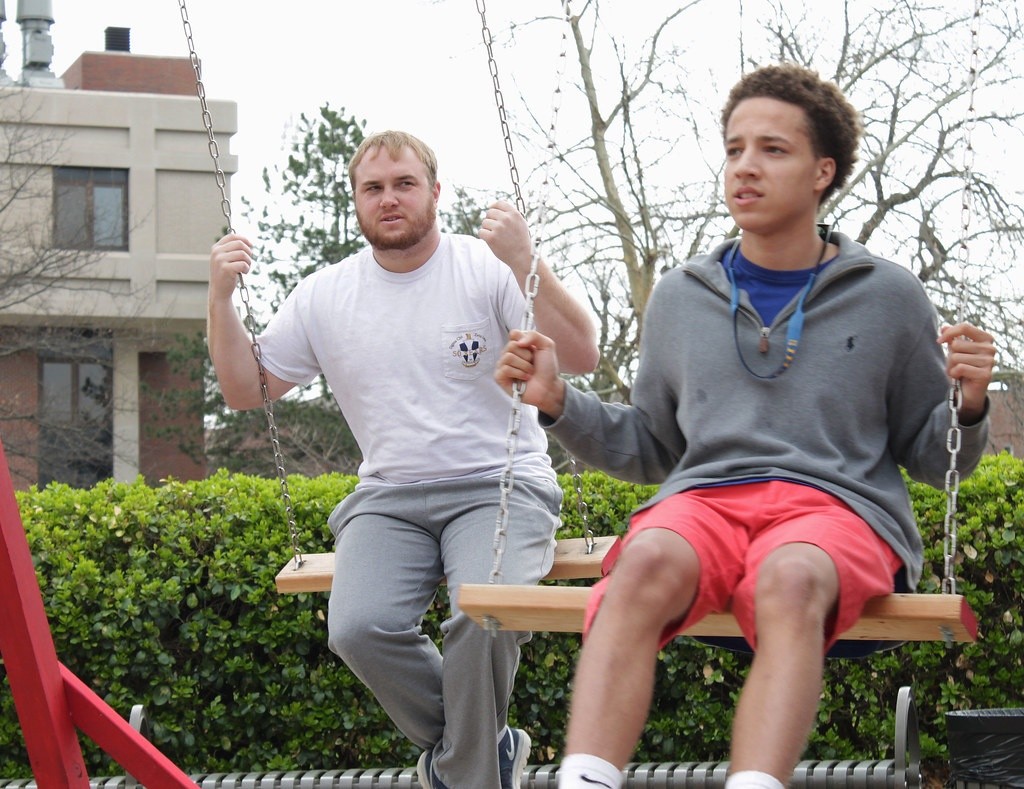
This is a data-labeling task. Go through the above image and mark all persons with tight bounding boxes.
[208,130,601,788]
[493,64,997,789]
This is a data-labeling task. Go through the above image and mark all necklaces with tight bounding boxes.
[728,223,831,379]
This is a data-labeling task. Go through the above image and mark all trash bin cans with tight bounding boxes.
[942,707,1024,789]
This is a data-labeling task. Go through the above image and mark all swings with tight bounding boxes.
[458,4,983,647]
[177,1,619,594]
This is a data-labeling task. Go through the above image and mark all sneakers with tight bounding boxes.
[417,750,445,789]
[498,723,530,789]
